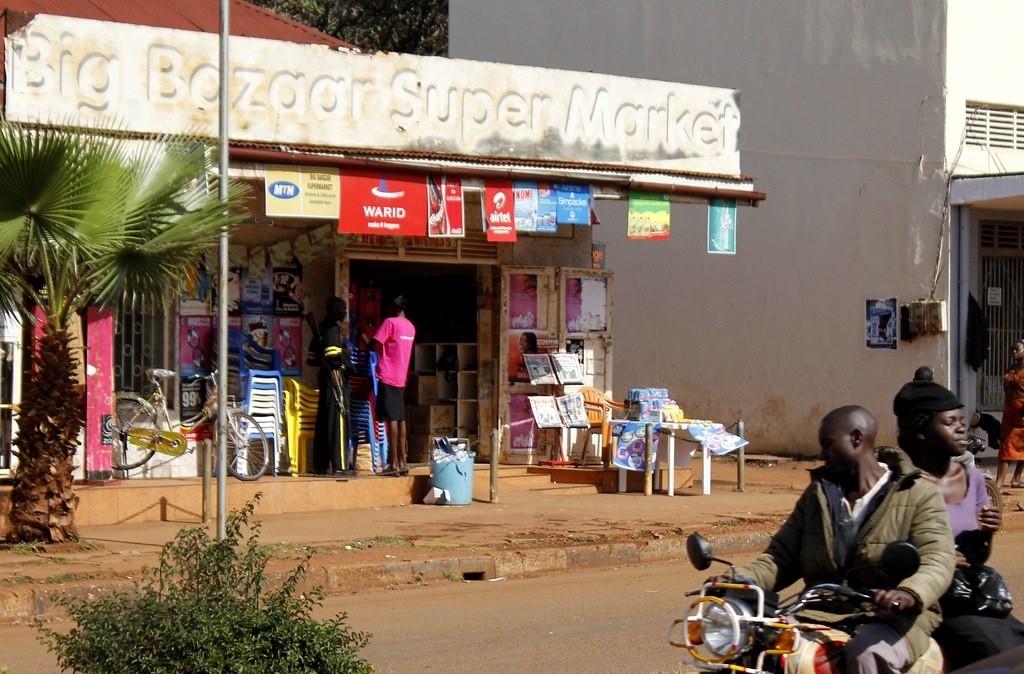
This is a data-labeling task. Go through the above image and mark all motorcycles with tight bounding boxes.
[667,531,945,674]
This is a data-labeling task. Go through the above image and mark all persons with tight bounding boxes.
[279,327,297,367]
[893,339,1024,674]
[306,295,416,477]
[273,274,304,304]
[187,329,207,367]
[517,332,541,384]
[703,405,956,674]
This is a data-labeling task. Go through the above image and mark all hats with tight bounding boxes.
[893,380,965,417]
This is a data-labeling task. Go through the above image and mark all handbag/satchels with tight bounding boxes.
[939,564,1013,618]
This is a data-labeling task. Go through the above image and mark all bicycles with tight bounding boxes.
[115,368,271,481]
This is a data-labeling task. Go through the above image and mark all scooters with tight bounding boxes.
[966,413,1003,506]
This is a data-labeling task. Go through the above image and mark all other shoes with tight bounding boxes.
[375,467,410,476]
[333,470,355,479]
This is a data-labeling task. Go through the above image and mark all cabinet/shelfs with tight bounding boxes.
[415,343,480,457]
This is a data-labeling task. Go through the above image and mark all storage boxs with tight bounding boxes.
[411,405,455,434]
[406,404,414,431]
[411,434,453,463]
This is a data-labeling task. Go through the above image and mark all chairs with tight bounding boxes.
[577,388,625,470]
[212,330,349,476]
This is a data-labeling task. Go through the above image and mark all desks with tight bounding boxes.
[610,420,723,495]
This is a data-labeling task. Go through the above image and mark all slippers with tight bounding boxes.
[1011,481,1024,489]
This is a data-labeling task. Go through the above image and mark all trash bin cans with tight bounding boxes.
[429,448,477,506]
